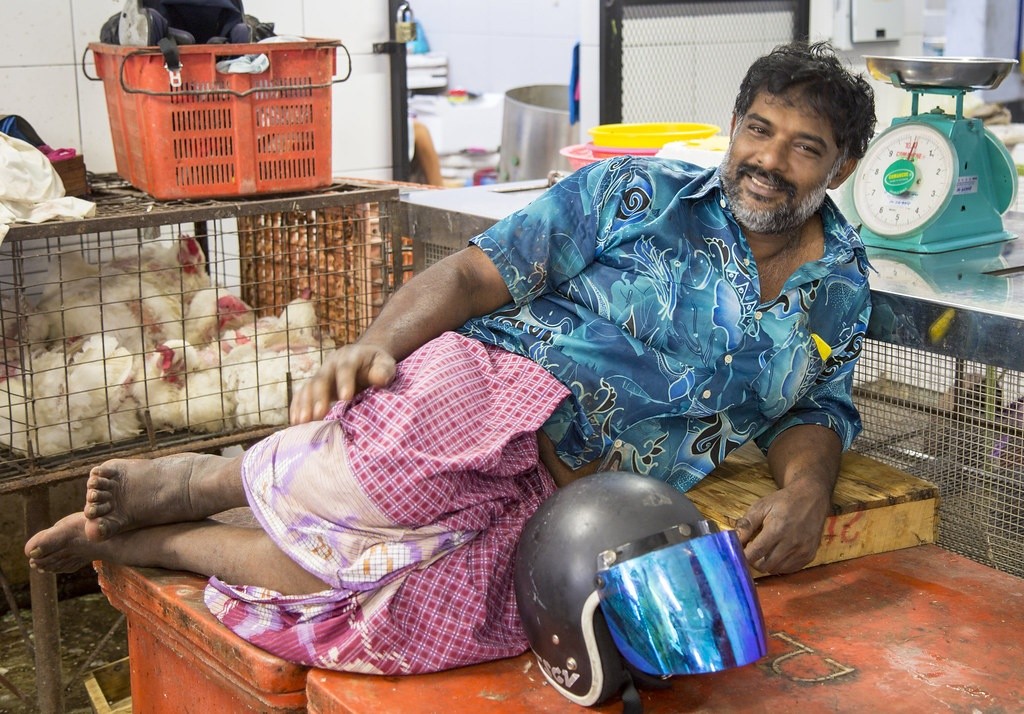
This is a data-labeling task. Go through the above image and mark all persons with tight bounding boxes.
[24,41,879,677]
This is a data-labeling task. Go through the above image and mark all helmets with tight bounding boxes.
[514,473,721,707]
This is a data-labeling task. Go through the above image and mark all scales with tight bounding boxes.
[852,53,1020,254]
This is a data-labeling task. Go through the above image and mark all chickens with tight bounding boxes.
[1,235,338,458]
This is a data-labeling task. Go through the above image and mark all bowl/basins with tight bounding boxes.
[559,121,722,172]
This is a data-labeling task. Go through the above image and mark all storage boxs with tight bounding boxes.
[81,33,352,200]
[88,559,1024,713]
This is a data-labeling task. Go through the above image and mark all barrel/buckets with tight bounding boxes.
[499,85,579,182]
